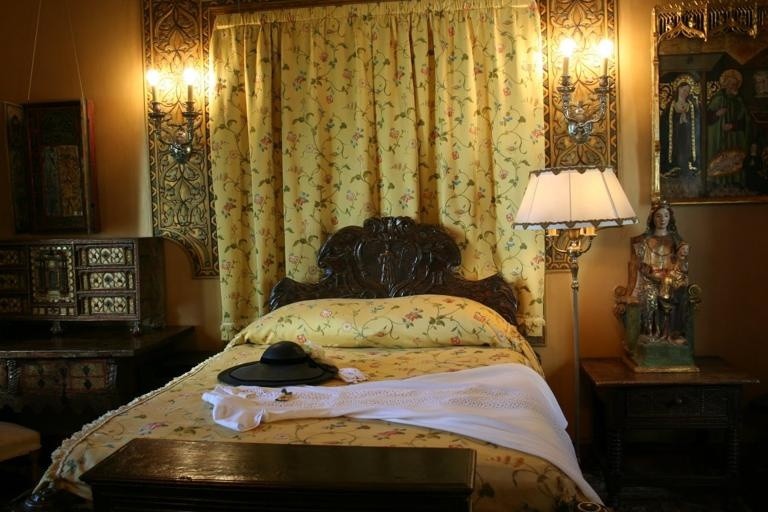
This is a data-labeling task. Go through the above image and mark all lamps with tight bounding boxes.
[509,164,640,483]
[557,35,616,144]
[146,67,203,165]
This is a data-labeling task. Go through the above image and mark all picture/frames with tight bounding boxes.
[650,0,768,206]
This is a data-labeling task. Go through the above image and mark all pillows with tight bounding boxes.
[222,294,543,375]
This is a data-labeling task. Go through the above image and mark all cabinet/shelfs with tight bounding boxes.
[0,235,168,336]
[1,327,195,433]
[77,436,477,512]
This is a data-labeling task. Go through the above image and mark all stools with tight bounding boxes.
[1,418,43,511]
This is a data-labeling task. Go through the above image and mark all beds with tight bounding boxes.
[29,218,610,509]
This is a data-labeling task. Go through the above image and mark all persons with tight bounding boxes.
[672,240,691,273]
[706,77,751,190]
[659,82,702,177]
[630,199,691,343]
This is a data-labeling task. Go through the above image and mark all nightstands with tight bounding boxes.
[582,356,762,509]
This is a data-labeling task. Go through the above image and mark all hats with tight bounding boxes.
[218,341,338,386]
